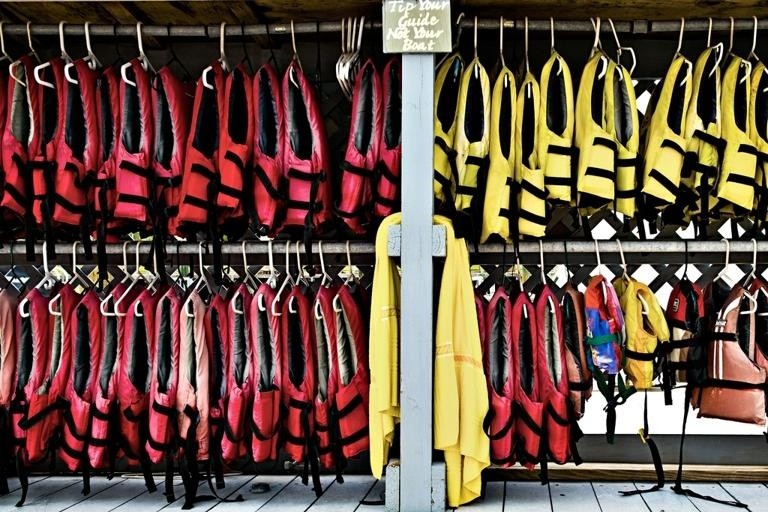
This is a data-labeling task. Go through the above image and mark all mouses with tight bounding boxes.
[471,238,768,318]
